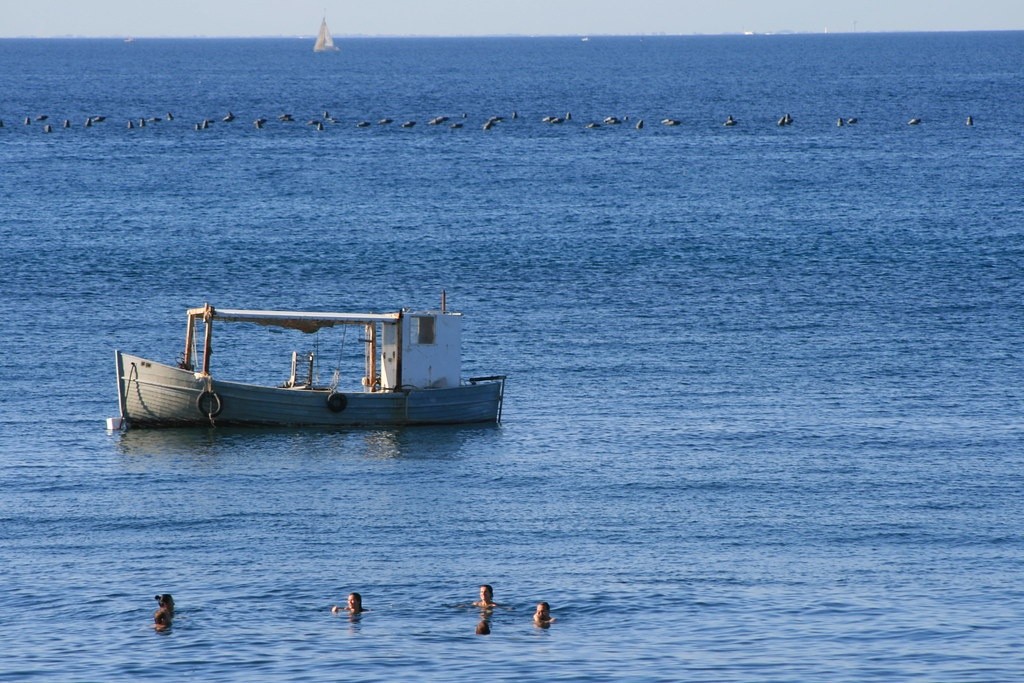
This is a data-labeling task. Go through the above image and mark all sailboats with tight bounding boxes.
[314,17,341,52]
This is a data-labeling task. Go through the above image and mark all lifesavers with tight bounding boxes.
[328,392,347,412]
[197,390,223,418]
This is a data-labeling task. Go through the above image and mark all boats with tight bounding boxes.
[115,286,507,426]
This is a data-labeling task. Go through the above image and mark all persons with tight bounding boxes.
[476,622,491,635]
[470,584,498,608]
[153,593,176,634]
[330,592,369,620]
[532,601,558,631]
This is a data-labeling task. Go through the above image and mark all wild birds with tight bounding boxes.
[25,115,71,133]
[586,116,643,129]
[907,117,921,126]
[128,112,173,129]
[483,112,517,129]
[85,116,105,127]
[967,116,973,125]
[428,112,468,129]
[279,114,295,122]
[305,110,341,130]
[357,118,415,128]
[195,112,235,129]
[542,112,572,123]
[254,118,267,129]
[661,119,681,126]
[724,115,737,127]
[777,113,793,126]
[837,117,857,127]
